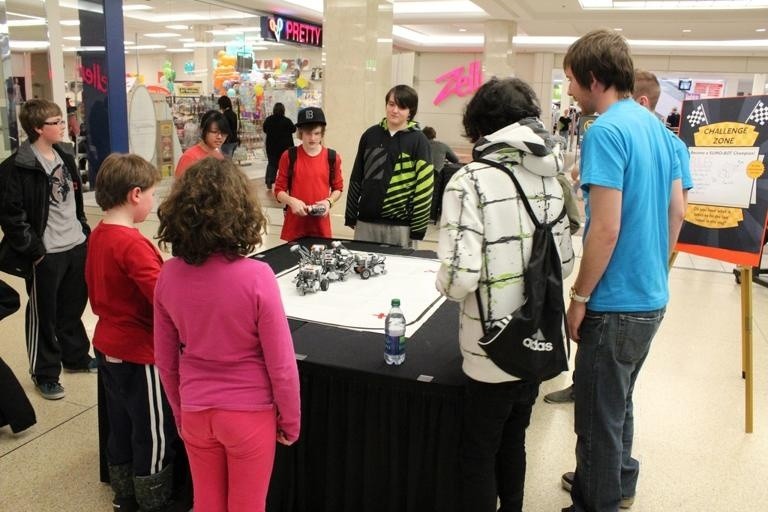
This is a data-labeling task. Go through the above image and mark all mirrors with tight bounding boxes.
[129,85,156,162]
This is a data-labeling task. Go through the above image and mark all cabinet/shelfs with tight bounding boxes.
[245,235,464,512]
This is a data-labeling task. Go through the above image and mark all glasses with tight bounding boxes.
[208,129,228,136]
[44,121,65,126]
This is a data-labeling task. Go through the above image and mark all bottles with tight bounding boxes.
[384,299,406,365]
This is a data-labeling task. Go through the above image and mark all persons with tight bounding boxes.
[275,106,344,241]
[13,77,25,103]
[175,110,227,182]
[0,280,38,434]
[422,127,458,223]
[665,107,680,136]
[544,68,692,404]
[154,154,301,512]
[437,76,574,512]
[86,152,178,512]
[263,102,297,190]
[344,84,434,250]
[558,109,572,152]
[219,95,239,159]
[508,77,581,429]
[0,100,96,401]
[560,30,685,512]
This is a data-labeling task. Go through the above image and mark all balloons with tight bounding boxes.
[126,50,318,119]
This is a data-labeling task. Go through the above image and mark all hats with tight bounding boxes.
[295,107,327,127]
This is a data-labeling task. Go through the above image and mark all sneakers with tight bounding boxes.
[562,473,634,509]
[63,357,98,373]
[544,383,577,405]
[34,383,66,400]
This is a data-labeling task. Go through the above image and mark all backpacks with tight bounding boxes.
[473,157,568,382]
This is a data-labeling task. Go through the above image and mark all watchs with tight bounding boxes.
[569,287,591,303]
[327,197,334,208]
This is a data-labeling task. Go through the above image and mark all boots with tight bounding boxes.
[133,461,192,512]
[105,460,138,512]
